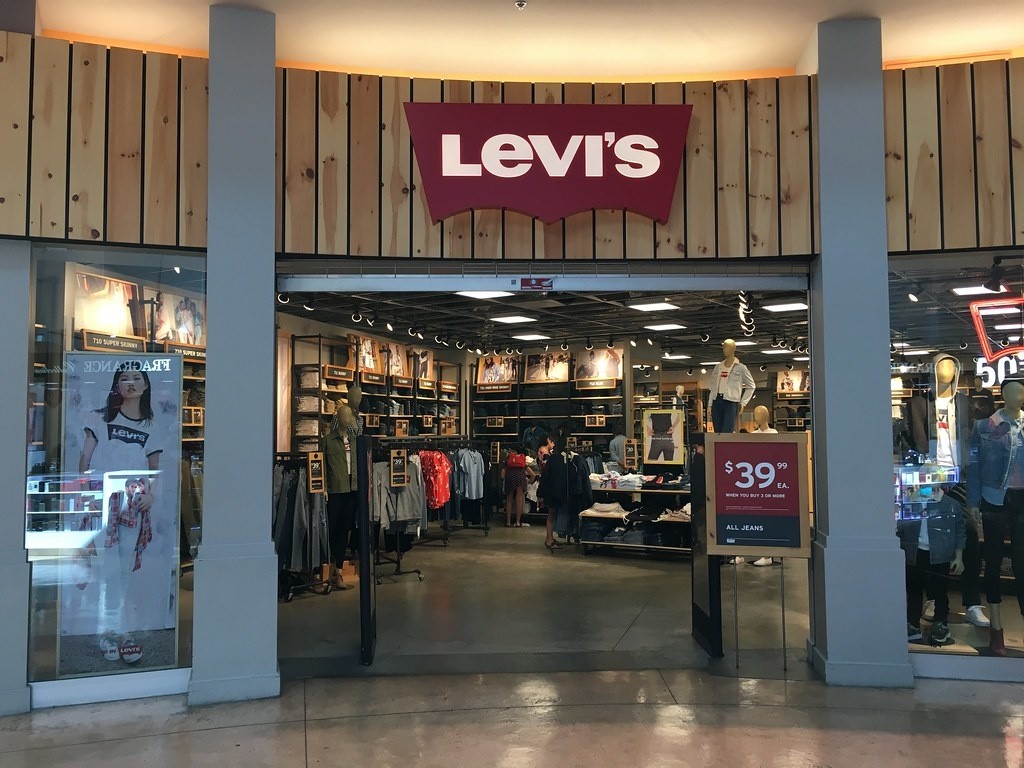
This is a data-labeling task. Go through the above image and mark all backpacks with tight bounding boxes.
[507,452,526,469]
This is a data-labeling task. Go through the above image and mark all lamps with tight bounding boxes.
[435,330,615,356]
[890,333,1024,368]
[278,293,429,339]
[909,286,924,302]
[984,258,1002,292]
[630,290,809,376]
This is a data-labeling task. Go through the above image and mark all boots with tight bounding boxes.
[991,628,1008,657]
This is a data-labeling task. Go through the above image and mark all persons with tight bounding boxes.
[779,371,794,391]
[894,351,1024,643]
[316,405,360,594]
[501,452,535,527]
[480,356,519,382]
[174,296,204,346]
[577,350,619,378]
[148,290,173,340]
[70,362,164,664]
[537,435,563,549]
[332,387,364,439]
[646,413,680,462]
[751,406,778,433]
[706,339,756,434]
[77,273,129,304]
[672,385,686,426]
[609,426,629,473]
[540,354,554,377]
[352,338,429,378]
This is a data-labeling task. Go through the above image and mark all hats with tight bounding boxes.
[1001,373,1023,392]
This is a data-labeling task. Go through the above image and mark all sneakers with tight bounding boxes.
[924,600,935,620]
[965,605,990,626]
[907,622,922,641]
[754,557,772,566]
[730,556,744,564]
[930,622,952,642]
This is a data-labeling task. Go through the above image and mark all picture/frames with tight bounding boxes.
[524,351,570,383]
[575,348,623,380]
[624,410,684,468]
[379,340,408,377]
[309,452,324,493]
[582,441,592,452]
[366,414,453,434]
[391,449,407,487]
[567,437,577,448]
[75,270,207,347]
[787,418,803,427]
[487,415,504,464]
[777,370,810,393]
[477,355,521,383]
[409,345,433,379]
[348,334,381,373]
[182,406,203,426]
[586,415,606,427]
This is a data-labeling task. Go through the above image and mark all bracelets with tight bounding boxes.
[147,493,155,502]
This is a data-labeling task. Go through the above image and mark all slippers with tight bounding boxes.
[505,523,511,527]
[516,521,521,527]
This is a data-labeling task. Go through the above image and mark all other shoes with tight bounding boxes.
[100,636,143,663]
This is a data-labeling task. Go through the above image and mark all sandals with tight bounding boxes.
[545,539,564,549]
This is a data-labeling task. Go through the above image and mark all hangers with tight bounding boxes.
[276,452,307,484]
[566,444,603,457]
[374,440,475,460]
